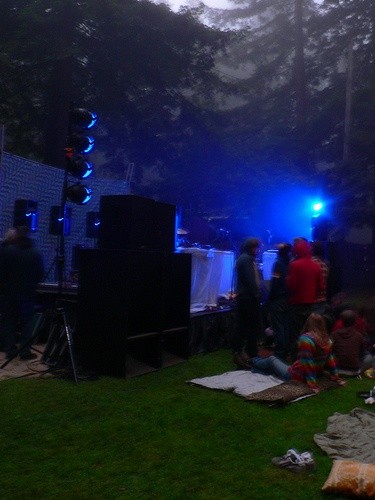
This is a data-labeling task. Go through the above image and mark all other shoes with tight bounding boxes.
[5,349,18,358]
[20,352,38,360]
[233,347,252,371]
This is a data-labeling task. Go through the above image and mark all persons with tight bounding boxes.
[233,236,260,367]
[261,235,375,377]
[3,224,46,362]
[232,312,347,397]
[0,227,21,246]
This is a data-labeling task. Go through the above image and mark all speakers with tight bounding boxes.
[11,198,37,231]
[48,205,72,236]
[86,211,97,237]
[96,193,178,252]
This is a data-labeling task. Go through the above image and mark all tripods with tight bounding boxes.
[4,141,81,385]
[40,236,67,286]
